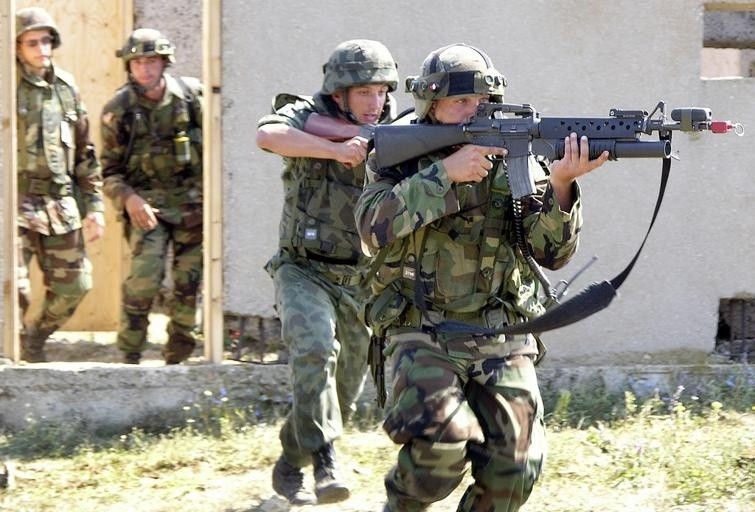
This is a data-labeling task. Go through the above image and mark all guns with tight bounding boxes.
[304,112,376,171]
[375,101,745,199]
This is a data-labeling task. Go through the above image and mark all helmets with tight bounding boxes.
[123,27,175,71]
[321,39,399,95]
[15,7,62,63]
[414,43,504,119]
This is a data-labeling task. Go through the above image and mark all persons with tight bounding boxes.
[16,7,105,363]
[100,28,202,366]
[255,39,400,506]
[352,42,609,512]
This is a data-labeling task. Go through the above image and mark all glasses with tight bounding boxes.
[18,35,54,47]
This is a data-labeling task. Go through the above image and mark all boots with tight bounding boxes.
[19,320,50,362]
[308,441,351,503]
[271,454,317,506]
